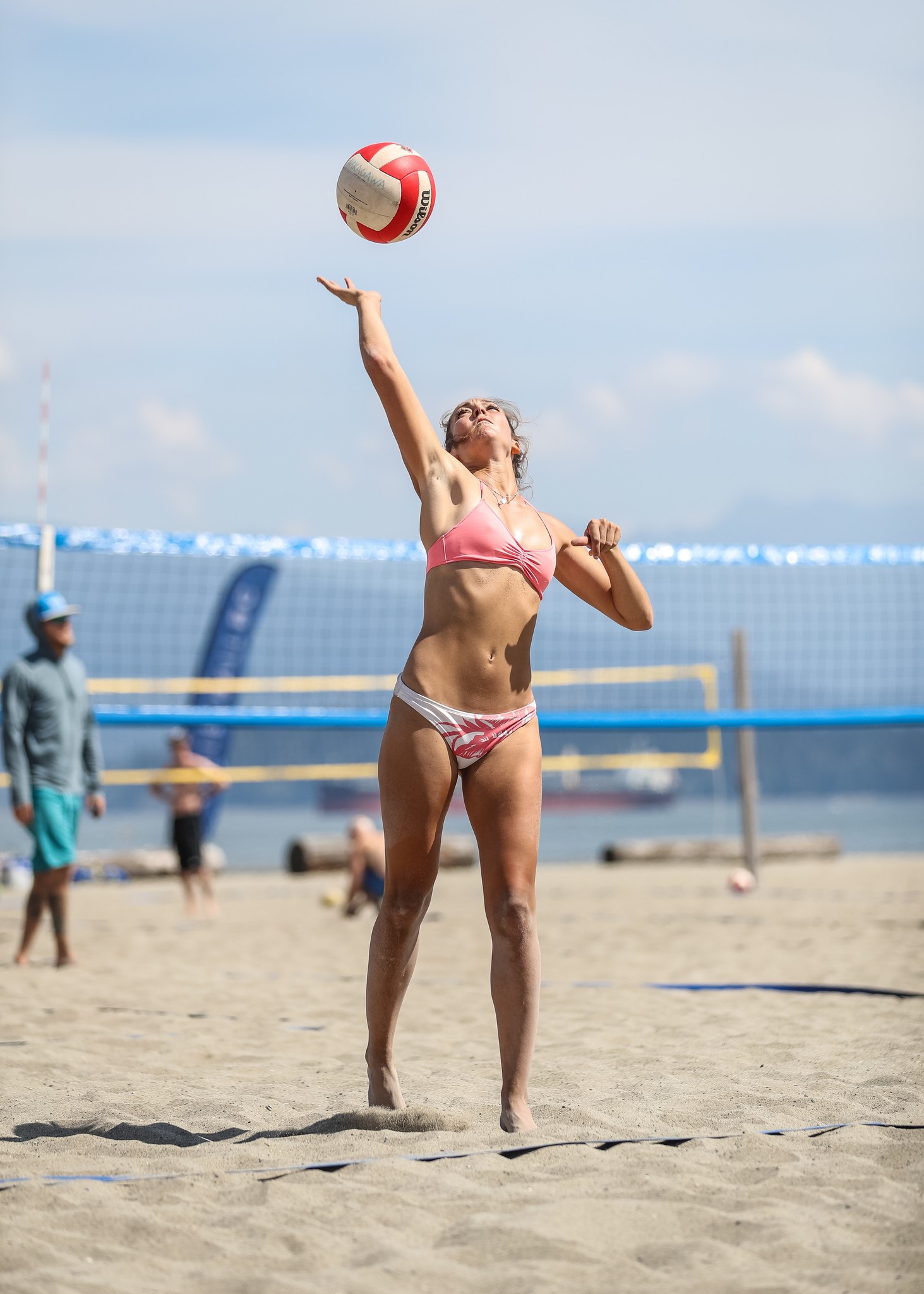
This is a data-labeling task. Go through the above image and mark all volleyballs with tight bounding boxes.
[336,142,434,244]
[321,890,342,908]
[726,869,755,892]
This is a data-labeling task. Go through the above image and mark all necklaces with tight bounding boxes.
[482,481,519,504]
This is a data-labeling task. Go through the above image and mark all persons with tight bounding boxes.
[345,816,386,914]
[149,728,230,904]
[0,592,107,965]
[316,274,657,1129]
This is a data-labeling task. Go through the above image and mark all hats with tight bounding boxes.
[26,592,81,627]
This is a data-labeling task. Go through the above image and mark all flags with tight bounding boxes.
[164,563,279,846]
